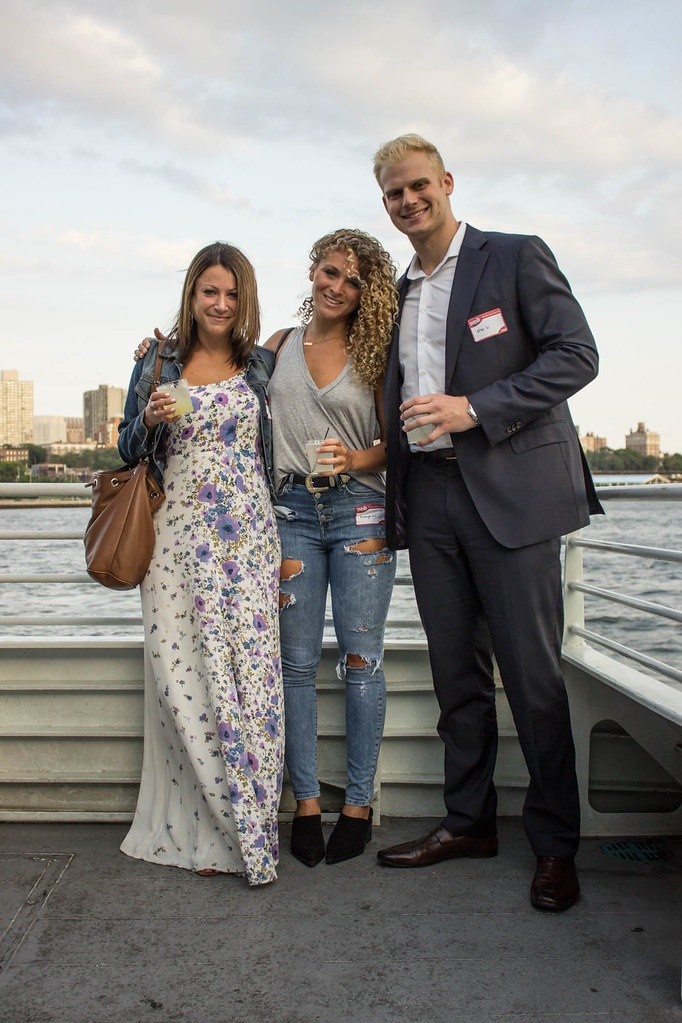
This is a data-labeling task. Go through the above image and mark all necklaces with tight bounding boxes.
[303,327,348,346]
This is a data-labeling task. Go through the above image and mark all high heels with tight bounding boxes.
[290,811,325,867]
[326,808,373,864]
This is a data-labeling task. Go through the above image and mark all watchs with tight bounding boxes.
[467,404,481,426]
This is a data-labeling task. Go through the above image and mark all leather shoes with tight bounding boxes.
[530,856,580,909]
[377,826,499,869]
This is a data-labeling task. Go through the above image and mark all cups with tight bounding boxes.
[404,414,434,444]
[156,378,194,419]
[304,439,333,473]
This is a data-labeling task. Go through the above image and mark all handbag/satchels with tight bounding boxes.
[83,460,167,591]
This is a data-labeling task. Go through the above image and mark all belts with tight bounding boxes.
[413,448,454,462]
[286,473,351,493]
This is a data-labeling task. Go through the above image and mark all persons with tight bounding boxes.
[373,131,606,912]
[133,229,399,867]
[117,242,286,886]
